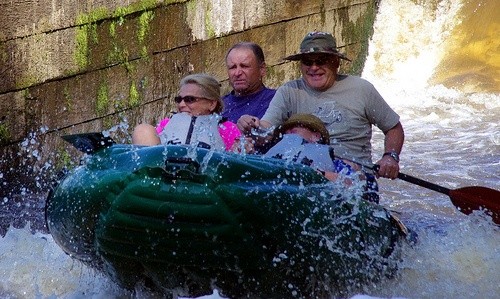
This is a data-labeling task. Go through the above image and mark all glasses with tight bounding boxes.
[174,95,212,104]
[300,59,334,67]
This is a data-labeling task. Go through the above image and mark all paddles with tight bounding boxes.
[372,165,500,225]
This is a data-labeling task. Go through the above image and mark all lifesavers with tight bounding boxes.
[264,134,336,172]
[159,112,226,153]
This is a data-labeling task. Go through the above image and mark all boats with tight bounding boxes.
[46,130,415,298]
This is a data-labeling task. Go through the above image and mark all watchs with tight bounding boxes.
[383,152,399,162]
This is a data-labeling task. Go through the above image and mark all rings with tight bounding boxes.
[385,173,390,176]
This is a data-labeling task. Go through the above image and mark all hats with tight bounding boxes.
[283,31,352,61]
[282,113,330,146]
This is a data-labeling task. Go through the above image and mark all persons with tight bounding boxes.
[132,73,254,155]
[256,112,361,187]
[221,42,277,121]
[237,31,405,180]
